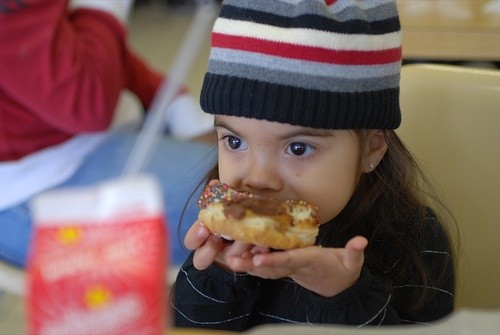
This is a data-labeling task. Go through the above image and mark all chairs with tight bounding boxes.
[393,63,500,309]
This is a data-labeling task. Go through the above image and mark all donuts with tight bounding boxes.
[196,180,320,250]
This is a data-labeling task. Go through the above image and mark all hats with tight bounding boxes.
[200,1,402,130]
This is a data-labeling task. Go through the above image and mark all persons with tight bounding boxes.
[1,2,218,270]
[168,1,455,331]
